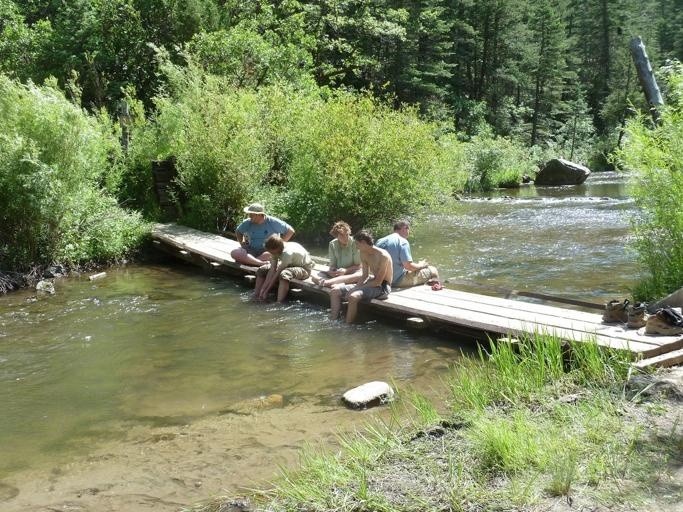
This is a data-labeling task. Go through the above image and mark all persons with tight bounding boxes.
[230,203,296,266]
[254,235,313,302]
[375,220,439,287]
[329,230,394,324]
[311,222,362,289]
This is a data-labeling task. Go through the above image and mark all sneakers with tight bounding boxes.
[646,315,683,336]
[602,300,629,324]
[627,302,648,328]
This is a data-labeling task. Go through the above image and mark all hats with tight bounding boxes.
[244,203,265,215]
